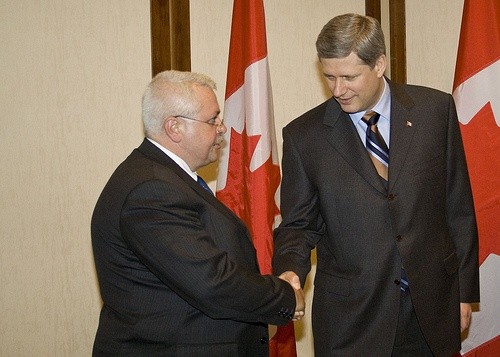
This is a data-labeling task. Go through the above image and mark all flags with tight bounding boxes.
[216,0,298,357]
[451,0,500,356]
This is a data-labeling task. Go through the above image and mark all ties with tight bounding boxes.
[360,111,410,294]
[197,174,214,197]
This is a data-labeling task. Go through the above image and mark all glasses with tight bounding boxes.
[173,115,225,135]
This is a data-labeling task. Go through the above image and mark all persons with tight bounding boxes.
[91,69,305,357]
[268,14,479,357]
